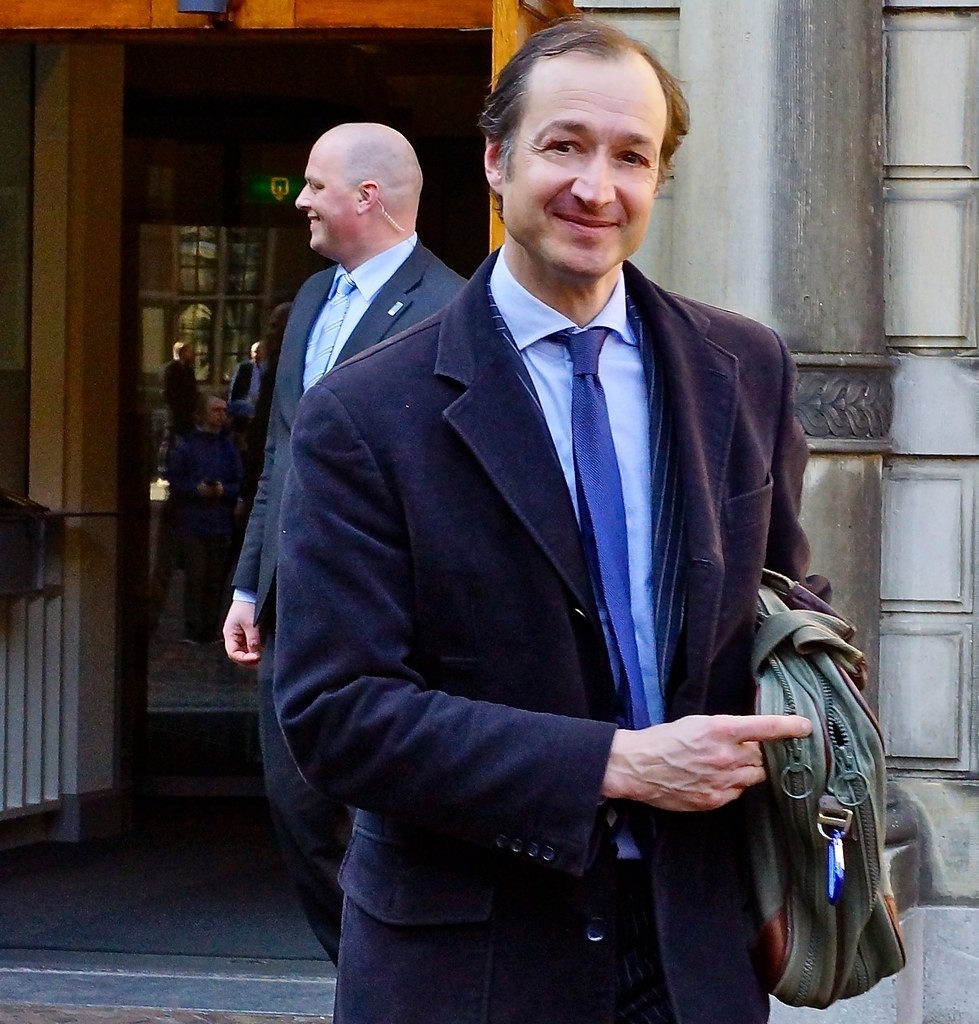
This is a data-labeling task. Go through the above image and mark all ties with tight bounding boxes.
[301,274,355,390]
[544,328,649,733]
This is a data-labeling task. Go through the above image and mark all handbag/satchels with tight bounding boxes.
[751,568,906,1010]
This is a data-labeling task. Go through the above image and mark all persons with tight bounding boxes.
[222,123,472,967]
[157,337,203,448]
[272,18,832,1024]
[243,302,293,642]
[165,395,243,647]
[227,342,268,446]
